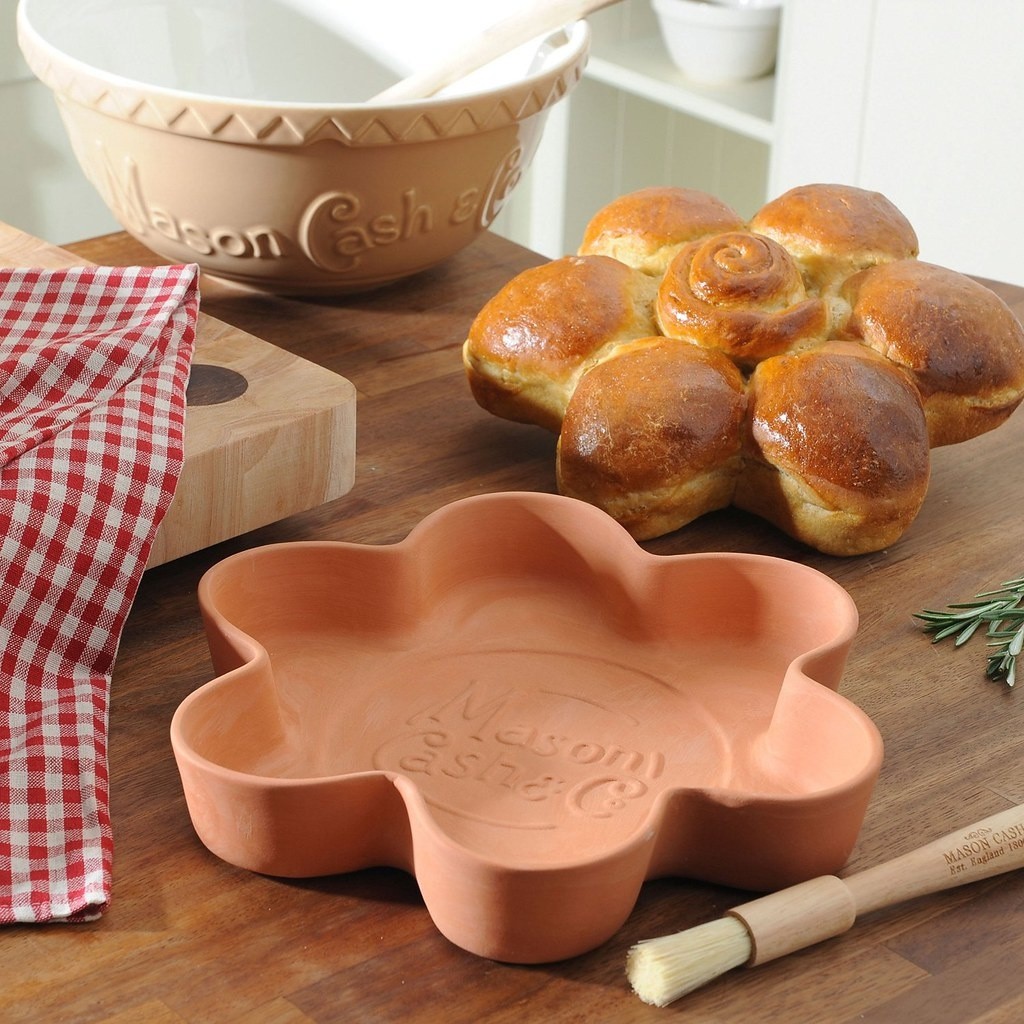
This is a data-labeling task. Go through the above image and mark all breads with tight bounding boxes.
[462,186,1024,558]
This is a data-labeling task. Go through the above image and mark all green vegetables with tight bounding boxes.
[910,572,1024,689]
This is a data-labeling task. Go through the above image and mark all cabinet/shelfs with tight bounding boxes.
[292,0,1024,286]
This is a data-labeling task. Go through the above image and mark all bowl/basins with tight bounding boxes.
[15,0,591,301]
[652,0,781,84]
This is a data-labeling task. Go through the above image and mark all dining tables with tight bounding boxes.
[3,227,1024,1024]
